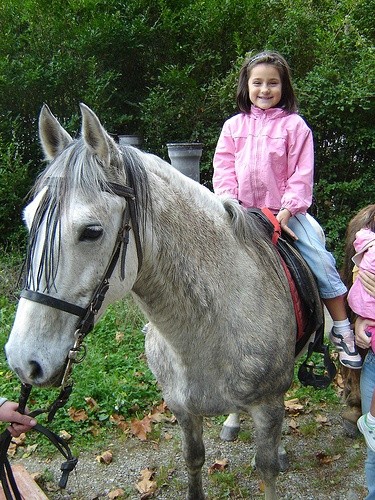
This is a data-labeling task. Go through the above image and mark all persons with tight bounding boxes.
[212,50,365,369]
[0,398,36,439]
[349,263,375,500]
[347,207,374,452]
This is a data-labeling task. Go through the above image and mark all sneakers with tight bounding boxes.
[356,413,375,454]
[329,328,364,369]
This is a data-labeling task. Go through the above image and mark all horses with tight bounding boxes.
[5,103,315,500]
